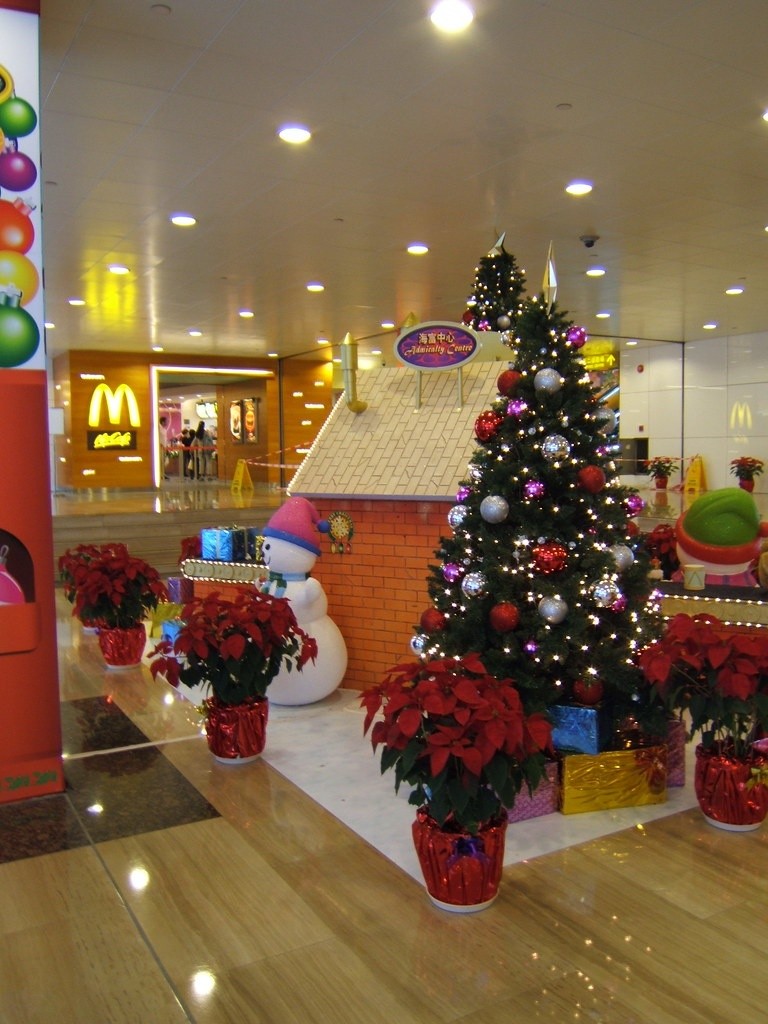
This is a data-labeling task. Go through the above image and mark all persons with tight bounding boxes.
[158,417,170,479]
[189,421,213,481]
[182,429,191,477]
[185,429,199,480]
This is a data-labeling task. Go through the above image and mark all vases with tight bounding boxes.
[81,616,106,635]
[203,694,270,765]
[654,477,668,489]
[96,623,146,670]
[740,478,754,492]
[411,805,509,913]
[694,735,767,831]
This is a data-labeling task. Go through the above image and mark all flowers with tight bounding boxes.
[640,613,767,761]
[68,557,172,629]
[58,542,131,601]
[729,456,764,480]
[178,537,203,566]
[358,654,557,836]
[643,457,679,482]
[646,522,680,580]
[147,591,319,704]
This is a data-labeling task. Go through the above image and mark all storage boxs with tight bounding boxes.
[162,617,187,647]
[151,601,188,638]
[554,714,668,815]
[201,523,259,563]
[482,761,554,824]
[167,577,194,606]
[647,718,686,787]
[548,694,612,756]
